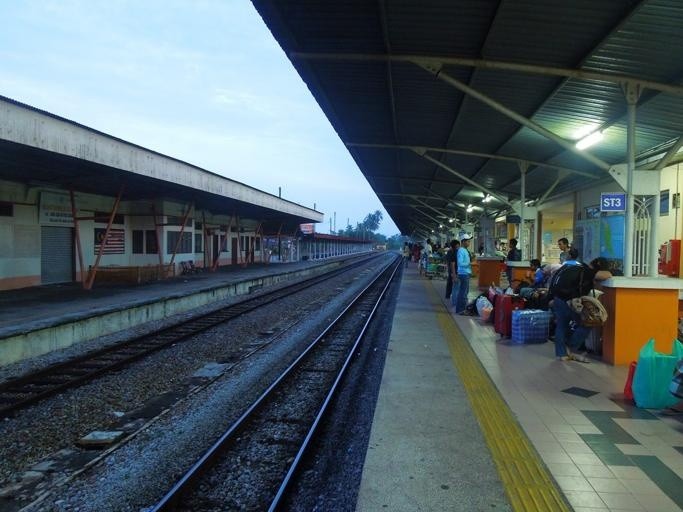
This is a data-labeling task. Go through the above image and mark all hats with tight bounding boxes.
[460,234,473,242]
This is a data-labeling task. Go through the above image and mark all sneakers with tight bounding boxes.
[443,297,473,316]
[558,351,592,363]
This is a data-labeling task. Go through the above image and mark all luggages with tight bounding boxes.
[492,293,525,335]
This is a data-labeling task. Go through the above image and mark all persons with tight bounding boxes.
[444,239,460,300]
[402,242,411,269]
[557,237,571,264]
[504,237,520,288]
[550,247,581,274]
[550,256,614,364]
[455,233,473,316]
[514,259,545,295]
[408,238,450,278]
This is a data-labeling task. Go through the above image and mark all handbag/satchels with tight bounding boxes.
[475,296,493,321]
[576,295,608,330]
[510,306,551,346]
[631,338,683,409]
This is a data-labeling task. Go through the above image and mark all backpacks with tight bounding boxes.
[550,263,586,300]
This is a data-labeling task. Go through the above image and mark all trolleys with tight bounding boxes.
[424,253,446,280]
